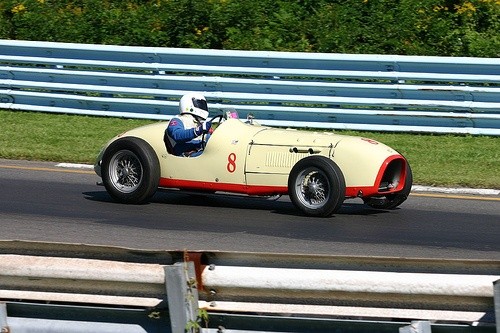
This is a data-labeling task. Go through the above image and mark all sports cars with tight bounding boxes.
[94,116,415,214]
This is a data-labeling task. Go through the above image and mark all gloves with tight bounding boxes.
[195,122,211,135]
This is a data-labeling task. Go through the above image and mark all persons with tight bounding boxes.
[167,93,213,158]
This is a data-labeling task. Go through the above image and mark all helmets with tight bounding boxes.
[179,92,209,120]
